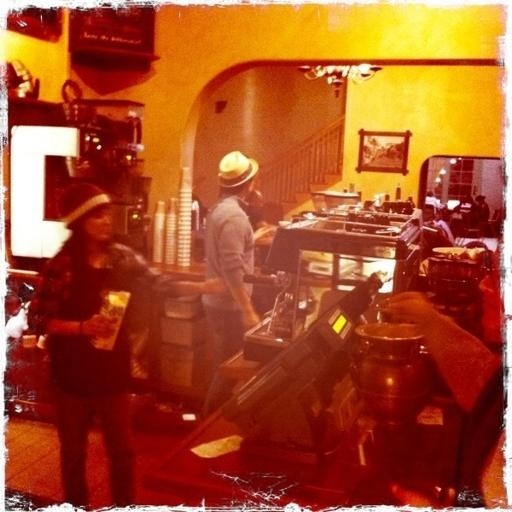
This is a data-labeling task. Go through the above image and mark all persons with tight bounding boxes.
[26,182,224,508]
[386,292,506,508]
[197,151,260,422]
[475,194,493,222]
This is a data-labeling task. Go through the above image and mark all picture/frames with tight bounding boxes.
[356,127,412,176]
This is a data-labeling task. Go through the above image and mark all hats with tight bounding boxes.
[217,151,259,189]
[60,183,112,228]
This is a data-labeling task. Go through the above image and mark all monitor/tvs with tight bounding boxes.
[306,273,386,327]
[223,304,356,425]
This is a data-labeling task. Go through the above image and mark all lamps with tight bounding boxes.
[296,62,383,98]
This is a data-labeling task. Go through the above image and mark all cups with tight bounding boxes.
[150,167,192,269]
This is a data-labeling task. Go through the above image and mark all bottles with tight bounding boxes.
[385,183,414,206]
